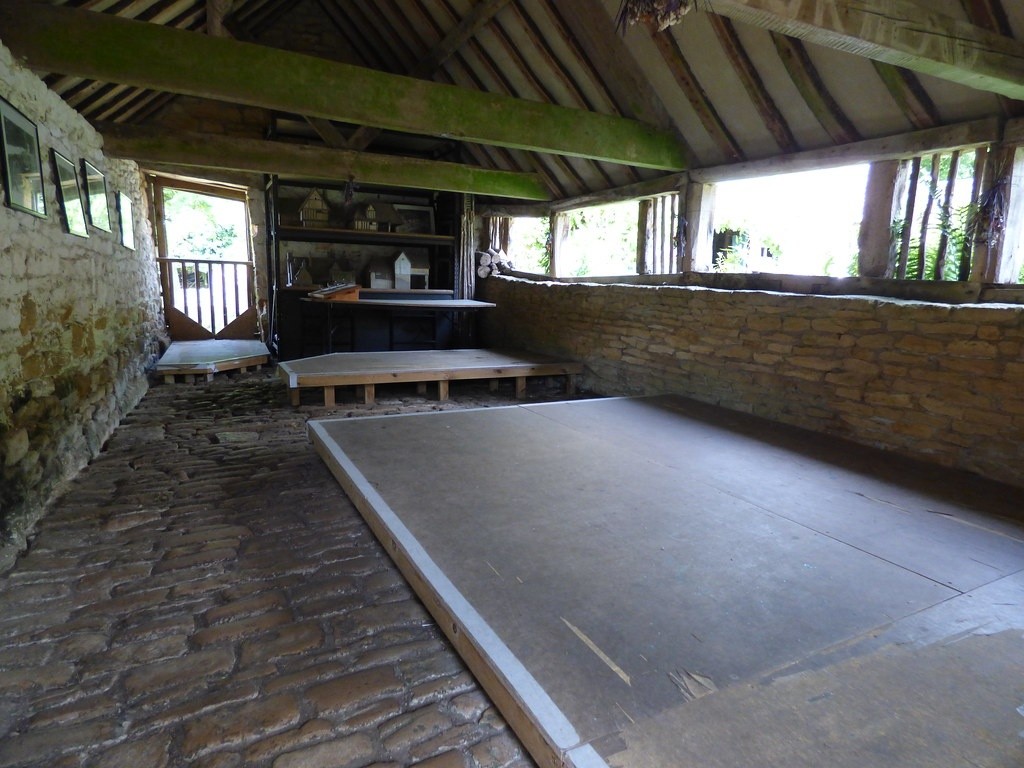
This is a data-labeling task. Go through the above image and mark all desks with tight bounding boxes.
[300,298,498,355]
[157,339,270,385]
[275,345,585,408]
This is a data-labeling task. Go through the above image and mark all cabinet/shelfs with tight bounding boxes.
[266,109,463,357]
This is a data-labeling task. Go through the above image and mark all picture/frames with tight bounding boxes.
[117,190,135,250]
[82,157,111,233]
[388,204,435,233]
[0,95,49,221]
[49,146,91,240]
